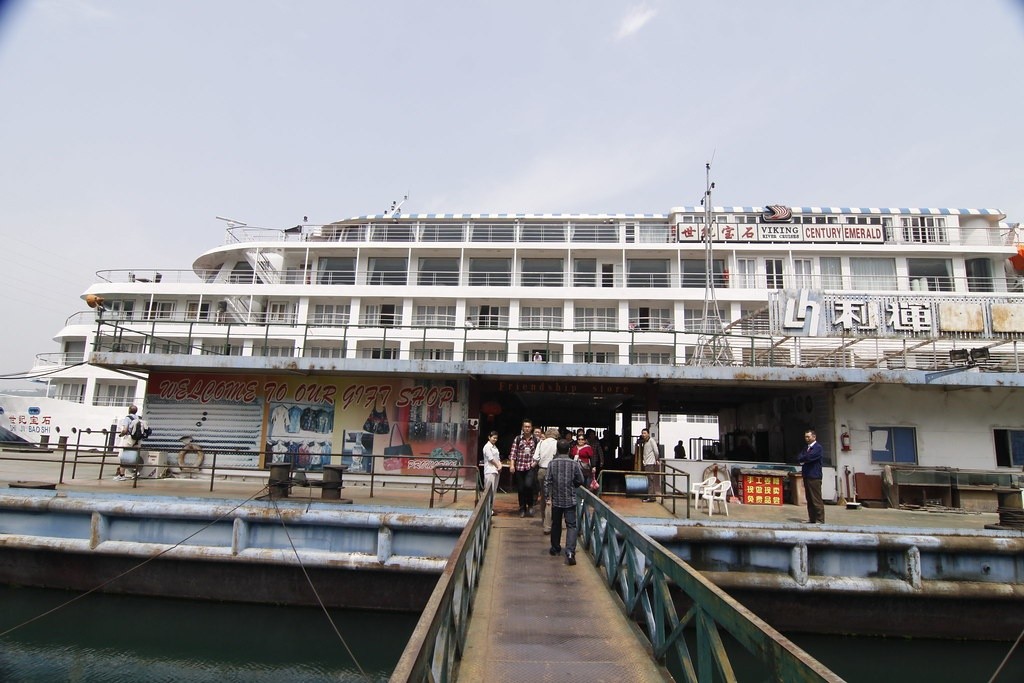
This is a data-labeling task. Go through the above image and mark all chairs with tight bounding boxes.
[701,480,732,517]
[690,477,717,510]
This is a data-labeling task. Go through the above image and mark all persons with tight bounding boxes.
[113,405,140,481]
[797,430,825,524]
[534,352,542,361]
[543,438,584,564]
[674,440,686,459]
[483,431,503,515]
[466,317,475,330]
[509,419,608,534]
[642,429,660,502]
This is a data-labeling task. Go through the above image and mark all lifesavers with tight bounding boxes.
[177,444,205,471]
[702,464,732,493]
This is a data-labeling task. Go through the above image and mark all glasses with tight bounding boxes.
[577,439,584,441]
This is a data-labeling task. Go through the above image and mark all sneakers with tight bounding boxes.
[131,469,140,480]
[113,474,126,481]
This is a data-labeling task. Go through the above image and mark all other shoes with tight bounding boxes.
[643,498,656,502]
[520,511,524,517]
[491,510,494,516]
[544,531,551,535]
[567,552,576,565]
[528,506,533,515]
[550,545,561,555]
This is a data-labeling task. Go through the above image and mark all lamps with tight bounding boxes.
[949,347,991,366]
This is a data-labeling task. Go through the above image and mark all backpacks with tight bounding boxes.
[128,415,144,440]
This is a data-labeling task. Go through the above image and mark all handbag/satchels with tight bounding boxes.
[590,473,600,492]
[573,475,582,488]
[384,424,415,470]
[430,441,463,471]
[365,393,389,434]
[142,426,152,438]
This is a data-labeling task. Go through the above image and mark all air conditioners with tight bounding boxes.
[124,451,168,479]
